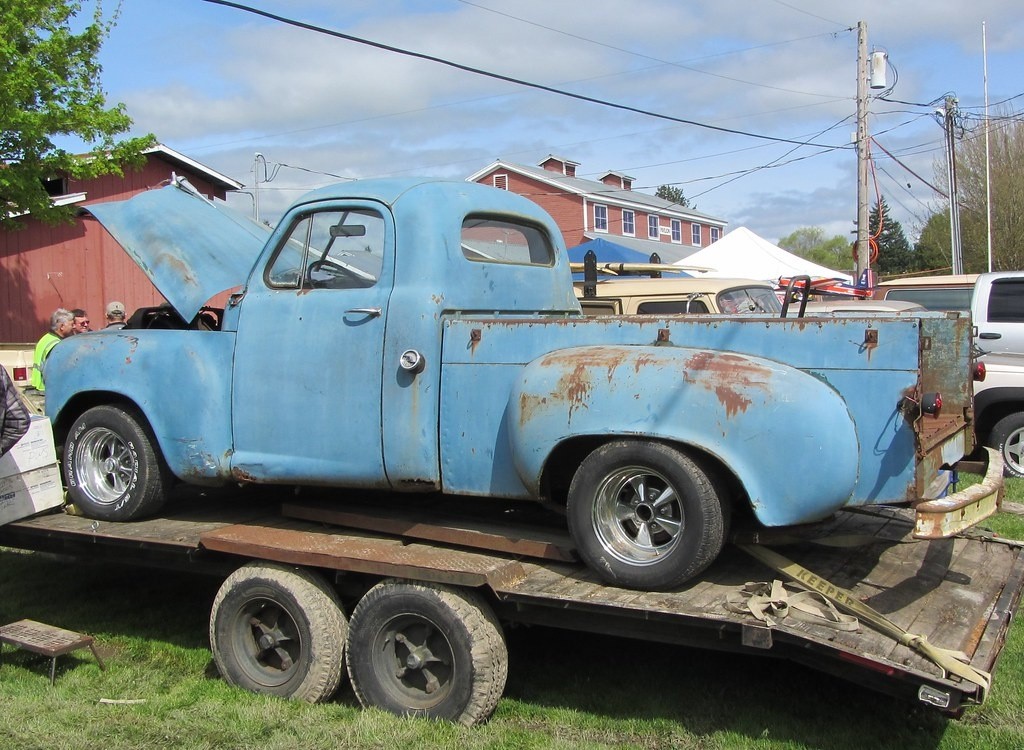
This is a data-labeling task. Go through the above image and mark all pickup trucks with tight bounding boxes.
[569,260,1024,480]
[38,169,1004,597]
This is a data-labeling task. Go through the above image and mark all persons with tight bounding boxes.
[32,309,75,391]
[0,364,31,459]
[68,309,92,337]
[105,301,126,330]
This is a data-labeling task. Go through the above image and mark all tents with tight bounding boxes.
[568,238,691,279]
[673,227,853,285]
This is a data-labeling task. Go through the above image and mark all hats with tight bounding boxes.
[106,302,125,317]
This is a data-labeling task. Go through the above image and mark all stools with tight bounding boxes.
[0,619,107,688]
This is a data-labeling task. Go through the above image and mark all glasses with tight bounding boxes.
[76,321,89,326]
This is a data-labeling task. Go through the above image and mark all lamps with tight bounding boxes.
[144,171,177,190]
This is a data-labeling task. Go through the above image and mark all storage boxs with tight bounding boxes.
[0,460,66,528]
[0,413,57,478]
